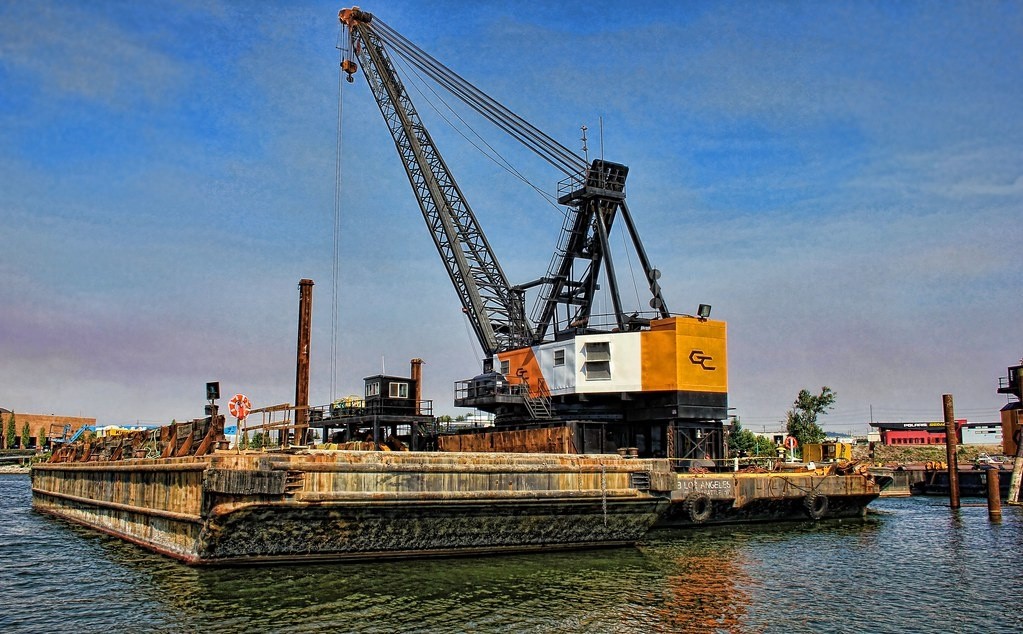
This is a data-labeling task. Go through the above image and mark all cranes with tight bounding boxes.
[336,5,728,466]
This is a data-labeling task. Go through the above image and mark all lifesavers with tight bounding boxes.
[785,436,797,449]
[804,489,829,520]
[684,492,712,525]
[229,394,251,419]
